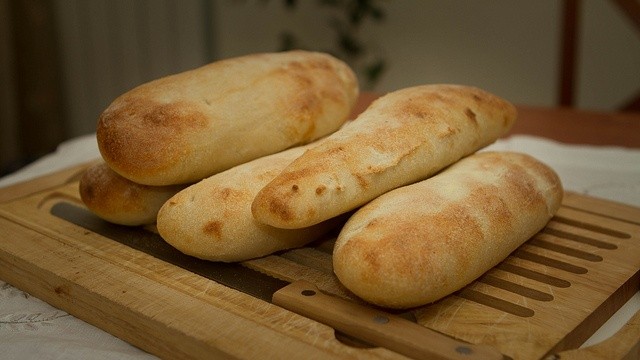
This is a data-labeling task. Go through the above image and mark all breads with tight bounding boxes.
[252,86,518,227]
[332,151,564,311]
[157,132,335,263]
[97,50,360,186]
[79,159,192,227]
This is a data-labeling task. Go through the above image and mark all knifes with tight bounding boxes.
[50,202,505,360]
